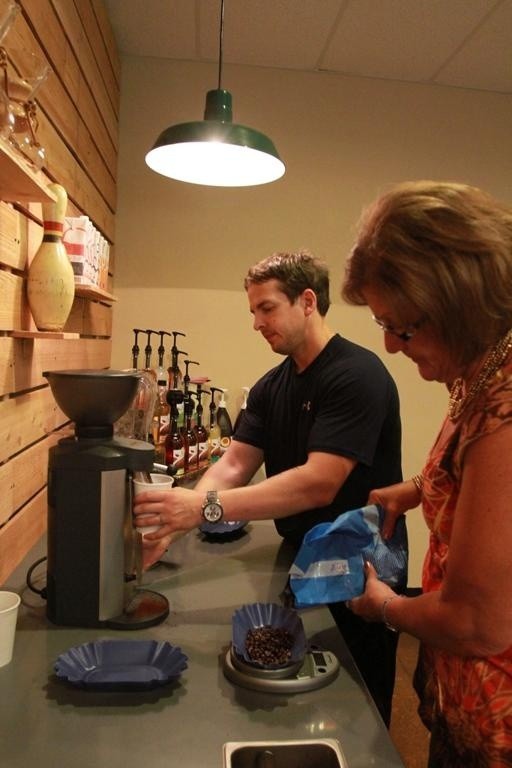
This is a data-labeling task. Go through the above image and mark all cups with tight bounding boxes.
[1,589,21,669]
[132,474,174,534]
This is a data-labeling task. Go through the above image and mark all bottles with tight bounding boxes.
[131,328,254,476]
[1,0,47,174]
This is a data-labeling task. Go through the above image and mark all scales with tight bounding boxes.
[221,644,340,694]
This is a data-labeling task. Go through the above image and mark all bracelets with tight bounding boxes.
[412,474,425,490]
[383,595,405,633]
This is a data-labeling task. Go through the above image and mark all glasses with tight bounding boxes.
[370,311,428,344]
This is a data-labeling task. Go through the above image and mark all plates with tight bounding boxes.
[199,519,251,535]
[232,604,311,666]
[54,641,189,688]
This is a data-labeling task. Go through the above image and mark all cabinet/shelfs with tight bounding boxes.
[0,138,119,340]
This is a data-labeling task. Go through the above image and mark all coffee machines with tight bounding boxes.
[39,365,173,631]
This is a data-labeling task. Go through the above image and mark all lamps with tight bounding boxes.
[144,1,287,188]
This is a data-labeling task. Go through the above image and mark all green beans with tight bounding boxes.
[244,624,295,666]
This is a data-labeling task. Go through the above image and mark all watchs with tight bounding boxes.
[202,490,223,522]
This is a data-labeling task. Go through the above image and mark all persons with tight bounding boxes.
[132,249,409,731]
[340,180,512,767]
